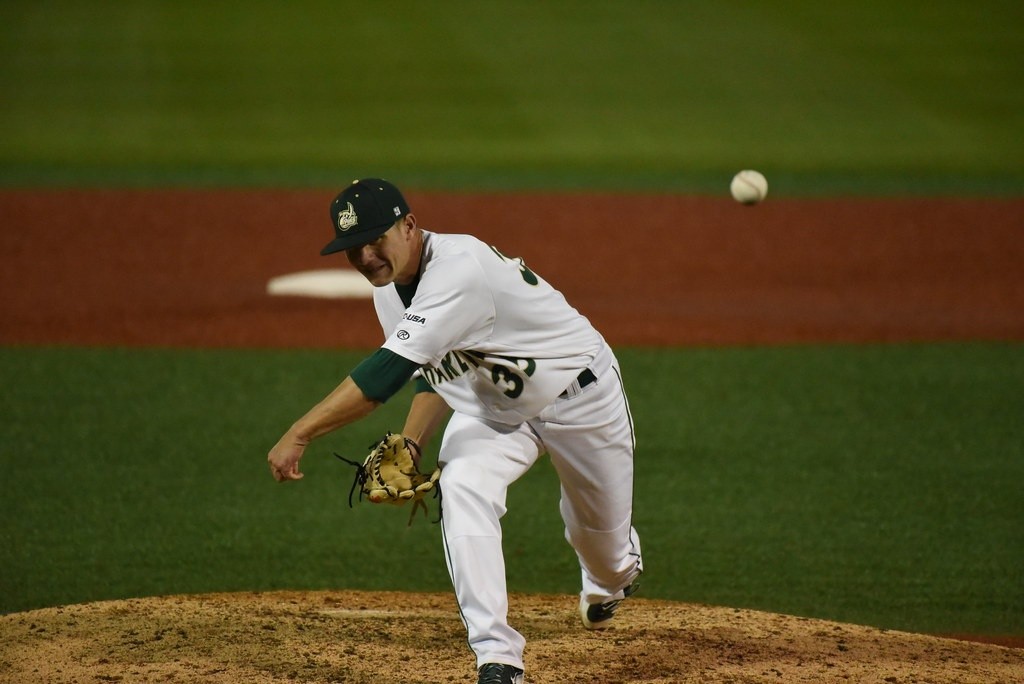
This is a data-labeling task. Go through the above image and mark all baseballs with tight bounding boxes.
[731,170,768,205]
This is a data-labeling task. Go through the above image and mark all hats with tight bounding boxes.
[319,178,410,255]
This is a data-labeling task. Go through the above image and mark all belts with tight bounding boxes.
[558,368,596,396]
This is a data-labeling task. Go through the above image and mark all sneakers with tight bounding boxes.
[579,582,640,631]
[477,663,524,684]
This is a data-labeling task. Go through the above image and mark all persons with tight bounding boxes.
[269,178,643,684]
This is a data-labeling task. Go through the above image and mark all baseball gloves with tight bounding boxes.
[357,433,441,505]
